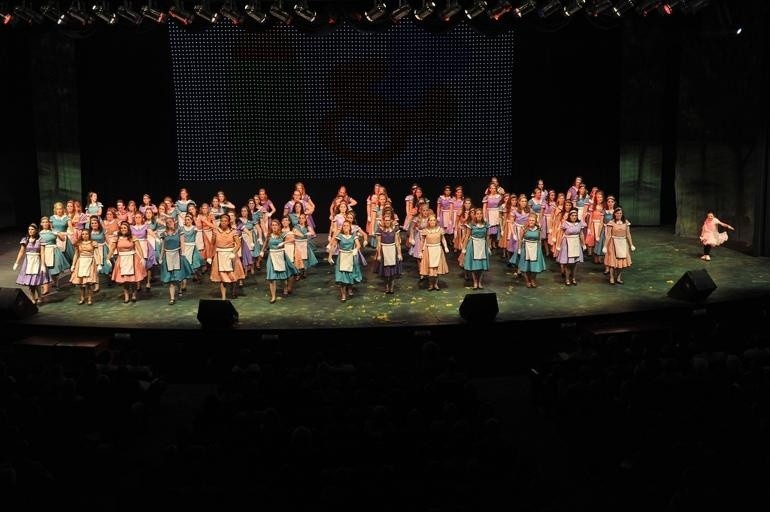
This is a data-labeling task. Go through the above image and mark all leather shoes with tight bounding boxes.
[427,283,434,290]
[87,297,92,306]
[608,277,616,285]
[478,281,484,289]
[123,297,130,303]
[390,289,394,295]
[473,281,478,290]
[531,280,537,288]
[36,296,49,303]
[256,263,262,270]
[77,298,84,305]
[93,287,101,293]
[181,286,188,291]
[178,290,183,298]
[587,252,593,258]
[526,281,532,288]
[231,291,237,299]
[146,283,151,289]
[340,295,347,302]
[167,298,176,305]
[269,297,276,303]
[239,282,244,288]
[347,291,355,296]
[385,286,390,294]
[295,274,302,281]
[603,269,609,276]
[136,287,142,291]
[572,279,578,286]
[565,280,572,286]
[302,273,309,279]
[131,294,137,302]
[616,278,625,284]
[434,284,441,291]
[284,290,289,298]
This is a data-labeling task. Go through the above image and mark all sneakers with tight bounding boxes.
[705,254,712,261]
[701,254,706,259]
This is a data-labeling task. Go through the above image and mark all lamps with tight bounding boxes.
[141,5,163,22]
[41,2,65,24]
[488,0,512,20]
[514,0,536,18]
[563,0,586,16]
[92,4,118,25]
[459,291,499,322]
[269,5,290,22]
[0,287,38,319]
[414,0,435,21]
[465,0,487,20]
[169,5,194,27]
[389,3,411,24]
[194,4,218,23]
[15,7,32,24]
[118,5,143,25]
[640,1,662,18]
[293,4,316,22]
[612,0,634,17]
[667,268,719,301]
[364,2,386,22]
[68,6,93,26]
[588,0,609,17]
[540,0,562,17]
[221,6,242,24]
[196,297,239,329]
[664,0,680,14]
[440,0,460,21]
[244,3,267,24]
[0,8,11,24]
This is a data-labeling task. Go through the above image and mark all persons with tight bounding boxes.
[411,204,441,282]
[594,196,624,275]
[64,200,82,245]
[330,195,344,215]
[437,186,456,246]
[216,192,236,214]
[102,208,122,278]
[292,214,318,281]
[556,208,587,286]
[283,190,318,239]
[497,192,516,258]
[418,215,449,291]
[74,201,90,231]
[49,203,75,291]
[371,194,395,248]
[260,219,301,304]
[329,186,357,220]
[374,214,403,294]
[601,206,635,285]
[185,213,216,283]
[528,187,549,255]
[89,215,106,235]
[454,198,480,262]
[584,188,605,255]
[458,210,488,282]
[139,194,158,215]
[326,213,368,283]
[485,178,506,195]
[37,217,70,291]
[700,212,735,261]
[178,212,204,290]
[197,203,217,267]
[89,220,116,294]
[409,203,439,273]
[531,180,548,199]
[247,200,268,271]
[376,202,399,229]
[400,198,434,248]
[366,184,398,232]
[404,188,430,229]
[375,204,401,261]
[329,200,352,220]
[508,194,533,277]
[461,208,492,290]
[373,209,403,276]
[13,224,53,313]
[227,211,254,287]
[541,191,558,256]
[279,215,304,295]
[164,198,186,228]
[345,210,357,225]
[175,188,197,233]
[85,192,103,229]
[328,220,363,303]
[185,203,223,279]
[369,187,399,237]
[126,201,146,225]
[115,200,133,226]
[236,204,264,279]
[258,189,276,230]
[585,191,610,264]
[253,195,268,256]
[328,204,355,242]
[155,202,172,240]
[482,183,506,249]
[143,208,165,283]
[129,212,163,292]
[159,218,194,305]
[548,194,569,259]
[405,185,429,216]
[207,214,246,300]
[106,221,147,303]
[286,202,307,231]
[498,194,522,258]
[573,184,593,212]
[509,214,547,288]
[69,228,102,305]
[553,199,580,276]
[566,177,588,212]
[295,182,316,228]
[210,198,227,226]
[450,187,480,253]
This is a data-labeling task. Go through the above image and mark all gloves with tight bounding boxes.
[582,221,588,227]
[487,248,493,256]
[277,242,284,248]
[556,246,562,253]
[206,258,213,265]
[97,264,103,271]
[228,253,235,259]
[587,229,592,236]
[351,248,358,256]
[602,247,608,255]
[549,228,553,234]
[327,234,332,242]
[159,259,163,264]
[259,251,265,257]
[461,248,466,255]
[105,258,113,268]
[582,245,587,251]
[397,253,404,261]
[500,230,505,237]
[325,244,332,250]
[70,265,75,272]
[454,231,458,239]
[140,258,147,268]
[257,239,264,246]
[327,256,335,266]
[630,244,636,252]
[363,240,369,248]
[39,265,47,276]
[441,238,450,254]
[513,235,517,242]
[366,216,372,223]
[264,233,267,239]
[12,262,19,271]
[552,236,557,244]
[265,213,273,218]
[518,248,521,255]
[436,217,443,223]
